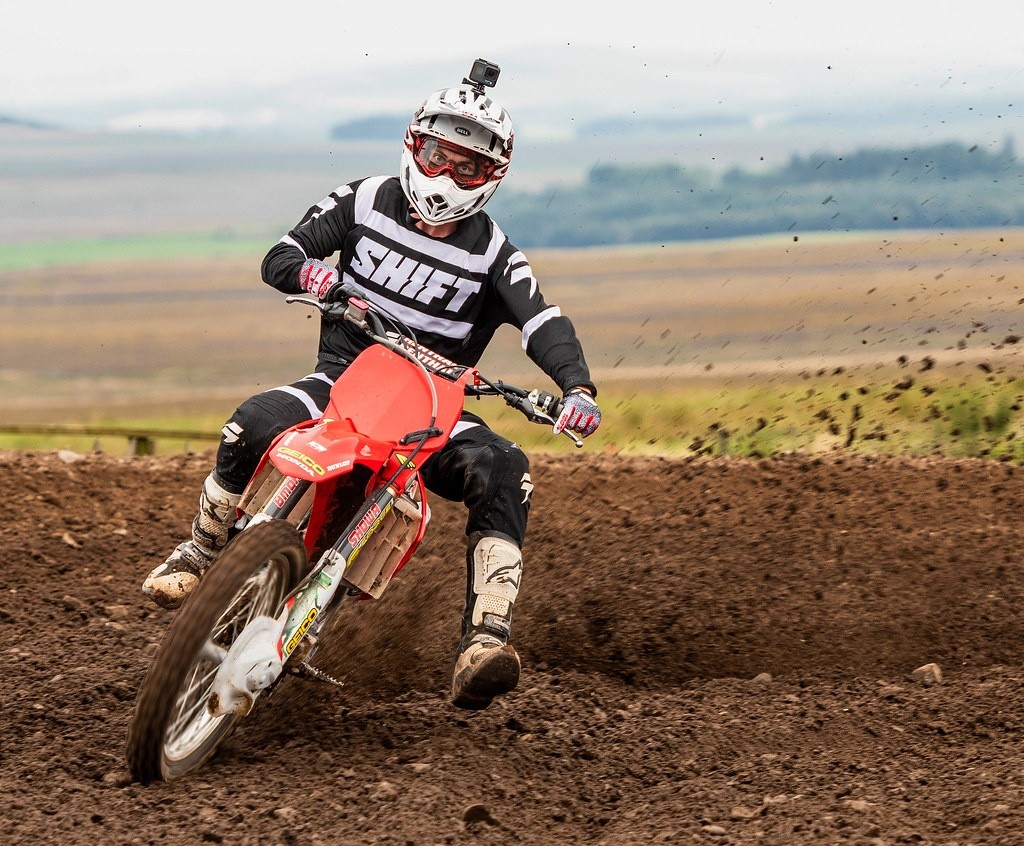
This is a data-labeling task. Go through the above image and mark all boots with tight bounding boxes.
[451,531,522,708]
[141,467,245,609]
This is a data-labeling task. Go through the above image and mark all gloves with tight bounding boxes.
[299,258,339,300]
[553,388,601,439]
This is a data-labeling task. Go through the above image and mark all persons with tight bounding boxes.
[142,82,600,711]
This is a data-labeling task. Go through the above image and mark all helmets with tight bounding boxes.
[399,84,515,226]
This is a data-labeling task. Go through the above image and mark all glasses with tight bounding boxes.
[414,137,495,190]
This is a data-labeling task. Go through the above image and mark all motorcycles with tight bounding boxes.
[123,273,587,793]
